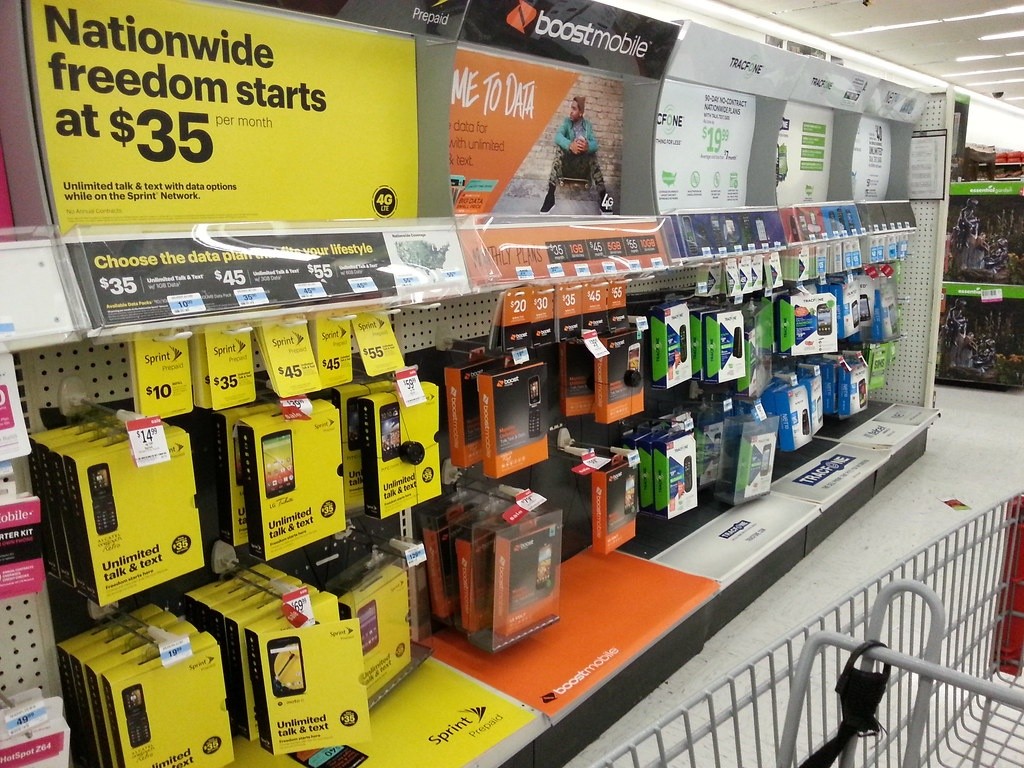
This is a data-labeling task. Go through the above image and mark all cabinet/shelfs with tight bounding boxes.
[1,0,554,768]
[652,15,817,637]
[762,47,889,560]
[412,0,714,768]
[936,175,1024,392]
[816,72,957,503]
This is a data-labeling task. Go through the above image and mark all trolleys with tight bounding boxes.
[585,488,1024,768]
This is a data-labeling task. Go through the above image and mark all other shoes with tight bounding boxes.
[599,204,613,215]
[540,194,555,214]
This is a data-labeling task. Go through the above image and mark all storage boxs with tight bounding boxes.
[0,237,921,768]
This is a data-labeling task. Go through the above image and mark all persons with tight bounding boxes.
[675,481,688,511]
[958,199,978,248]
[540,96,614,216]
[673,351,684,380]
[946,298,966,347]
[860,383,867,408]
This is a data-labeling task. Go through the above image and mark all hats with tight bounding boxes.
[574,96,585,114]
